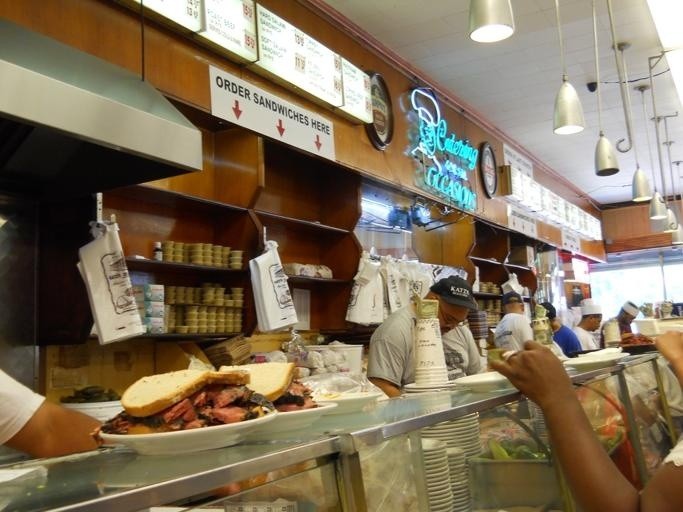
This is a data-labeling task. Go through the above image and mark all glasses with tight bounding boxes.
[438,307,464,327]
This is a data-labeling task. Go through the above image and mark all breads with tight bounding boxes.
[121,370,250,416]
[219,362,299,401]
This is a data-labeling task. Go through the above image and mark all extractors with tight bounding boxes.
[0,17,202,204]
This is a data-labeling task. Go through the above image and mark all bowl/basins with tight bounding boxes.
[173,255,184,262]
[217,319,225,325]
[230,262,243,269]
[234,300,244,307]
[185,305,200,312]
[225,319,234,325]
[166,285,202,304]
[226,307,234,313]
[231,251,243,257]
[476,297,502,312]
[192,260,204,266]
[183,243,190,263]
[212,245,231,268]
[168,305,183,326]
[217,325,225,333]
[164,254,174,262]
[164,248,174,254]
[225,325,235,333]
[192,249,203,255]
[231,287,243,293]
[168,326,175,334]
[479,279,500,293]
[203,244,212,250]
[201,293,215,300]
[226,313,234,320]
[231,293,244,301]
[198,325,208,334]
[204,261,213,265]
[200,305,208,312]
[202,286,215,293]
[224,301,234,307]
[235,308,243,313]
[207,313,217,319]
[215,293,223,298]
[234,325,242,333]
[224,294,231,300]
[234,319,243,325]
[198,319,207,325]
[203,256,213,261]
[215,287,225,293]
[201,298,213,305]
[188,325,198,334]
[185,312,198,318]
[190,243,203,250]
[207,325,217,334]
[174,248,183,256]
[217,313,226,319]
[198,311,207,319]
[208,307,217,313]
[231,256,242,262]
[164,240,174,247]
[234,313,242,320]
[184,318,198,326]
[174,242,184,249]
[214,298,224,307]
[191,255,203,261]
[203,250,213,256]
[207,319,217,325]
[175,325,189,334]
[217,309,226,313]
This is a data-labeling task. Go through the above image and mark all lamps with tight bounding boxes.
[664,117,683,244]
[466,0,514,43]
[591,2,619,176]
[552,1,585,135]
[647,59,678,234]
[639,89,668,220]
[619,48,652,204]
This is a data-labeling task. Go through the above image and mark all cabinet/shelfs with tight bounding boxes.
[0,345,679,511]
[0,0,607,397]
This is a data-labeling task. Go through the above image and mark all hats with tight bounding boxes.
[539,302,556,318]
[429,275,476,311]
[581,304,602,316]
[501,291,522,305]
[622,301,639,317]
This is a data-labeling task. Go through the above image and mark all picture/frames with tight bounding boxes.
[363,70,394,151]
[477,141,499,200]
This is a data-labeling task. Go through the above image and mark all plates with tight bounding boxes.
[403,382,457,389]
[564,352,630,372]
[445,447,472,510]
[314,395,383,416]
[455,372,507,390]
[98,410,278,452]
[421,412,482,456]
[528,399,549,447]
[407,438,454,512]
[254,401,338,433]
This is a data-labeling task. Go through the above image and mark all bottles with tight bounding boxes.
[153,241,163,261]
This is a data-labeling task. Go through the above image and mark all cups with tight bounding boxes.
[414,318,449,385]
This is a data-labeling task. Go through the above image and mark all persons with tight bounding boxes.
[492,291,534,367]
[602,302,641,348]
[576,304,604,350]
[538,303,582,360]
[496,329,683,510]
[364,276,485,398]
[0,362,108,460]
